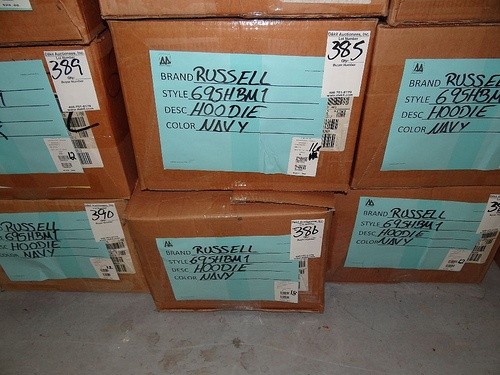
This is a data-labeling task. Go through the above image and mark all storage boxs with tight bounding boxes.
[0,0,500,314]
